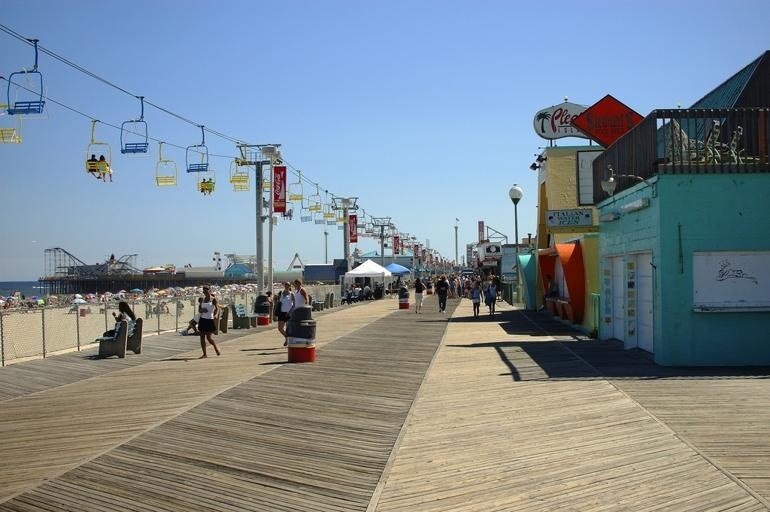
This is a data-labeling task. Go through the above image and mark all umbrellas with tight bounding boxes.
[383,262,411,286]
[344,259,391,301]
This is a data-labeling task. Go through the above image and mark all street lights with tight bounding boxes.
[509,183,523,303]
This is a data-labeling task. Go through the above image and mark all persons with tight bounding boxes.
[87,155,101,179]
[183,297,203,335]
[199,285,220,360]
[0,283,292,316]
[201,178,212,196]
[98,155,112,182]
[292,279,308,310]
[537,274,559,311]
[276,282,295,347]
[103,302,136,356]
[340,274,499,320]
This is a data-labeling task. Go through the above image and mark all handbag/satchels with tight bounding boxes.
[275,302,282,316]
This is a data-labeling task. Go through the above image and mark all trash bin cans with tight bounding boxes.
[81,309,88,317]
[254,296,270,325]
[286,305,316,363]
[399,288,409,309]
[427,283,432,294]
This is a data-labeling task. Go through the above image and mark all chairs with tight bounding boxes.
[663,117,744,164]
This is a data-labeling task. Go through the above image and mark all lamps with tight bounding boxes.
[601,163,657,198]
[530,155,547,171]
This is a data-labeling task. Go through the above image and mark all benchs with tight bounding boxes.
[231,304,259,329]
[307,292,334,311]
[95,318,143,359]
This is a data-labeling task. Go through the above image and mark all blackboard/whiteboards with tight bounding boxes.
[692,250,768,313]
